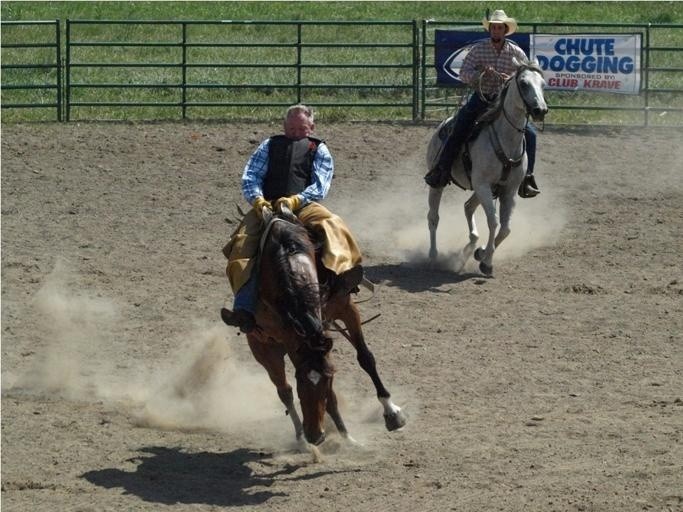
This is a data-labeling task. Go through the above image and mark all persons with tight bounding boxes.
[220,104,363,334]
[424,8,540,196]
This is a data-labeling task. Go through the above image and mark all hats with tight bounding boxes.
[483,10,517,35]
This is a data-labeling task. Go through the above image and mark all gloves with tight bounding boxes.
[274,194,301,212]
[253,196,273,220]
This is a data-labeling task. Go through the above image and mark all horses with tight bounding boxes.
[426,57,547,276]
[220,214,406,452]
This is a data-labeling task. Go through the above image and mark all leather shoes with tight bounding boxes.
[518,182,541,195]
[424,168,452,184]
[221,308,255,333]
[328,265,363,298]
